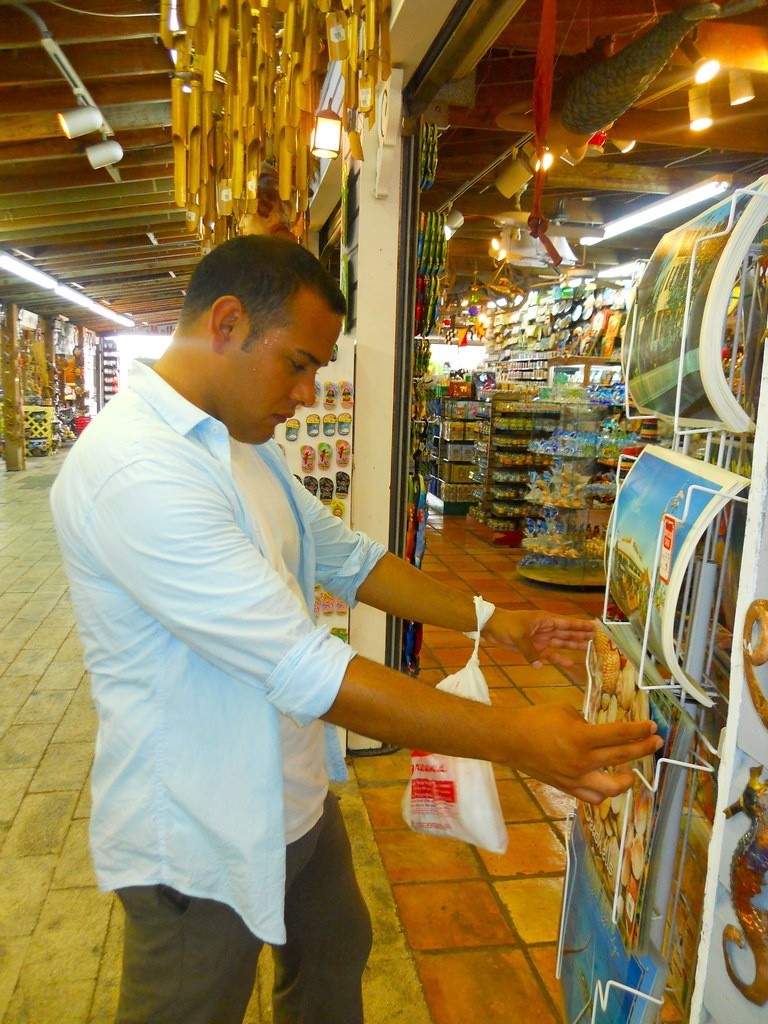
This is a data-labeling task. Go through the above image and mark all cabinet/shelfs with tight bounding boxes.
[408,270,675,591]
[555,174,768,1022]
[0,302,122,457]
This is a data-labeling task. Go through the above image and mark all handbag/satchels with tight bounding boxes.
[398,596,512,853]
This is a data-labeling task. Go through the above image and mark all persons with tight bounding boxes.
[48,235,665,1022]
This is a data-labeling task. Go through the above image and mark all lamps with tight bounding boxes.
[85,139,125,170]
[56,105,104,140]
[435,36,757,262]
